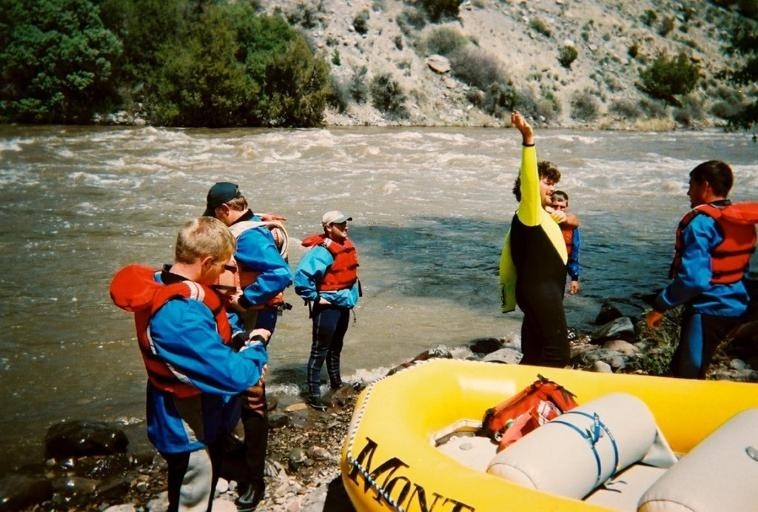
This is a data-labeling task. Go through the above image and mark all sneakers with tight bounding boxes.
[335,382,360,390]
[235,485,265,508]
[308,395,327,408]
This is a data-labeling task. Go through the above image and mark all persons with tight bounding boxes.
[644,158,758,381]
[547,190,579,297]
[108,215,271,512]
[199,179,294,512]
[292,210,361,410]
[498,110,580,368]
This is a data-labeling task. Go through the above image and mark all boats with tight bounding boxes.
[338,356,753,511]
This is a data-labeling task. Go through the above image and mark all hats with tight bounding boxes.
[203,182,240,215]
[322,210,353,227]
